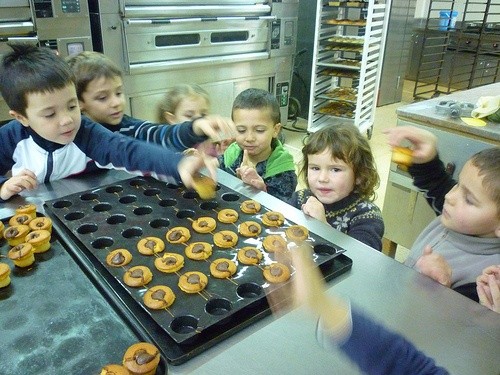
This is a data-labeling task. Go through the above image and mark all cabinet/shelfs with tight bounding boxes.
[307,0,393,140]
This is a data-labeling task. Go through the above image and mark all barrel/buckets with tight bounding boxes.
[437,9,458,31]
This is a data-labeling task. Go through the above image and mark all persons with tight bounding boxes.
[288,120,385,251]
[386,124,500,312]
[286,244,448,375]
[476,265,500,314]
[184,89,298,199]
[0,39,233,202]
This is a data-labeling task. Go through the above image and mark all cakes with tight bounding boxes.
[193,175,217,199]
[0,263,10,288]
[185,241,212,260]
[154,253,185,273]
[192,217,216,233]
[178,271,208,293]
[237,246,262,265]
[263,263,290,283]
[99,342,160,375]
[137,237,165,255]
[0,204,52,267]
[166,227,191,244]
[213,230,238,248]
[218,200,308,253]
[210,258,236,278]
[123,265,152,287]
[143,285,176,310]
[391,146,414,166]
[107,249,132,267]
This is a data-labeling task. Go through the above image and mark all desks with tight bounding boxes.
[403,25,500,89]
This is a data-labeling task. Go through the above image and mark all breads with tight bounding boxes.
[346,1,365,7]
[327,37,364,43]
[326,19,365,25]
[327,69,360,78]
[328,1,343,6]
